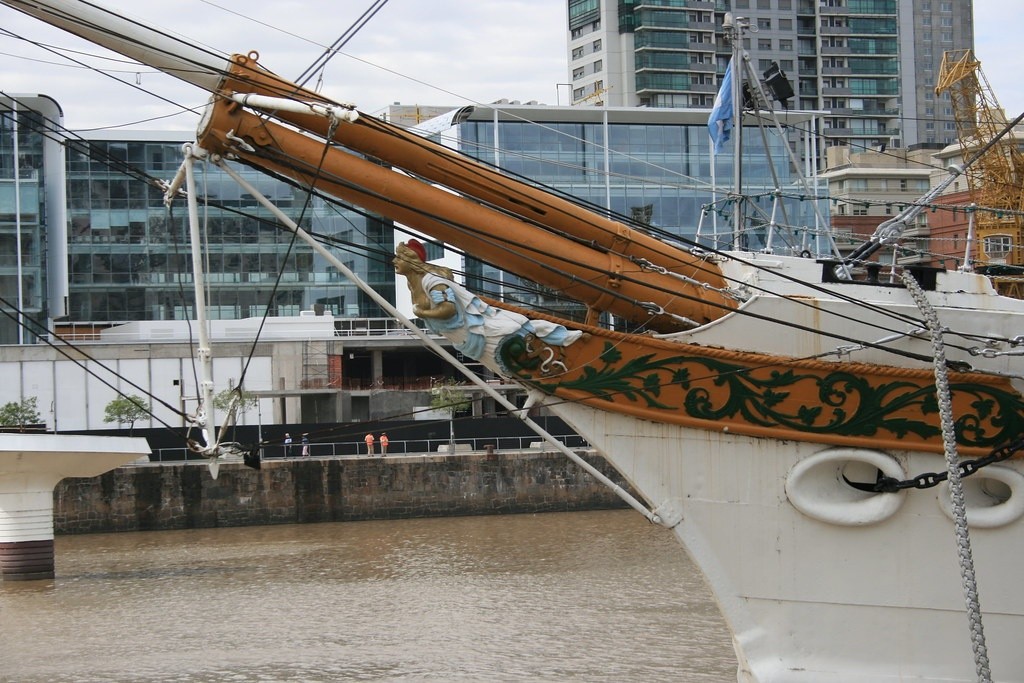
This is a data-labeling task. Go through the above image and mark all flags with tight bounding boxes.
[708,57,735,154]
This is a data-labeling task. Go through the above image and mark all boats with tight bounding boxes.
[0,0,1023,682]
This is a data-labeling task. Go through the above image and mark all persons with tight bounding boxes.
[301,433,309,456]
[284,433,292,457]
[392,241,582,370]
[364,433,374,457]
[380,433,388,457]
